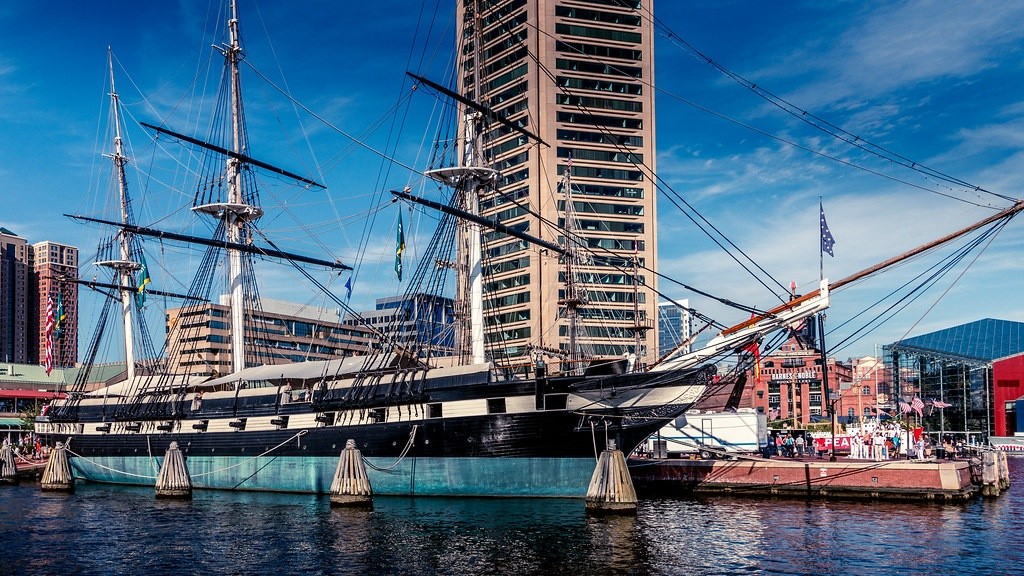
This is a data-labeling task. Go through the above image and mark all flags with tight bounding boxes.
[344,277,352,300]
[743,314,760,385]
[55,288,67,340]
[138,252,151,310]
[395,204,406,283]
[45,291,56,377]
[877,396,952,418]
[820,205,836,257]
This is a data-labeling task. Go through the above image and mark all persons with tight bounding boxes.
[14,435,44,463]
[776,433,814,458]
[852,430,931,461]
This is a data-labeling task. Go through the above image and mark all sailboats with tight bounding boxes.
[30,0,1024,505]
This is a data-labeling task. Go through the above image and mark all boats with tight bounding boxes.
[0,416,52,481]
[986,431,1024,455]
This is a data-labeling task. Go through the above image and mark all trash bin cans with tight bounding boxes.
[935,447,946,459]
